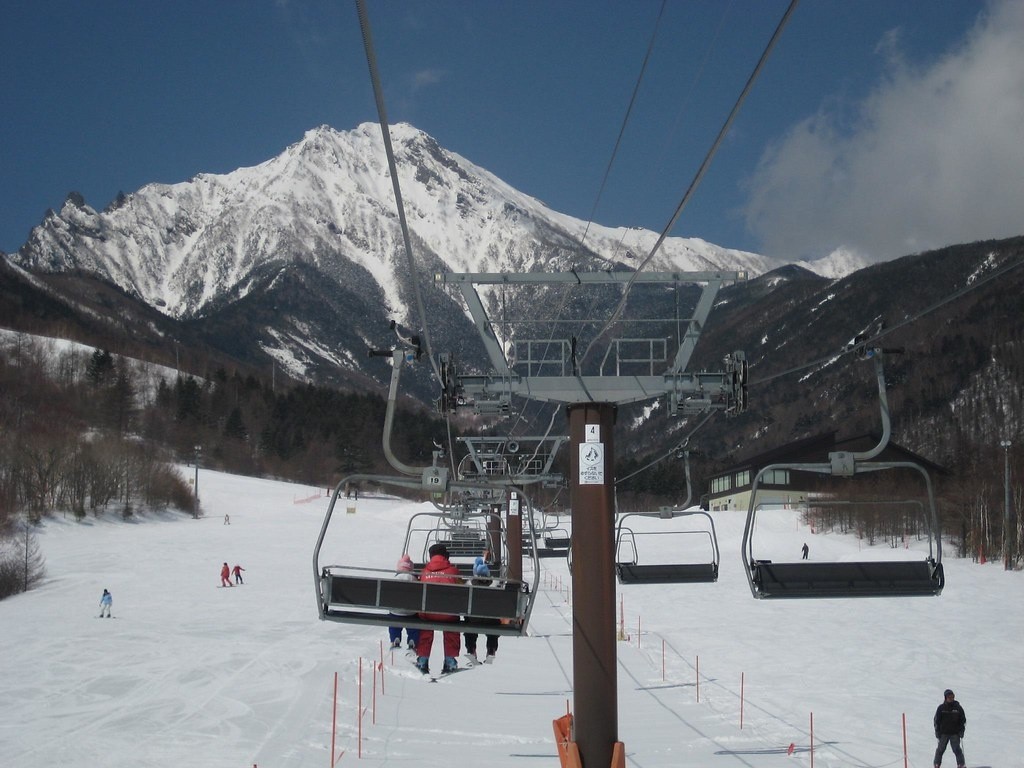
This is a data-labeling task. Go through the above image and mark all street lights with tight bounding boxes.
[1000,439,1011,570]
[194,444,202,519]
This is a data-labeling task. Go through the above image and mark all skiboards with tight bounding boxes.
[389,641,417,651]
[406,651,471,685]
[465,652,495,668]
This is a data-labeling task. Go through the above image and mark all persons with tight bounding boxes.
[99,589,112,618]
[802,543,808,559]
[220,562,232,587]
[464,556,500,664]
[934,689,966,768]
[338,488,358,501]
[412,544,462,674]
[224,514,230,525]
[389,555,420,649]
[231,563,246,584]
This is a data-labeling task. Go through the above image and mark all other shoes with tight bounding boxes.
[486,650,496,658]
[468,651,478,660]
[415,656,429,673]
[391,638,400,649]
[409,640,415,649]
[444,657,458,671]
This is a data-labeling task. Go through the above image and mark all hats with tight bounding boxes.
[473,556,490,577]
[397,556,414,573]
[429,544,450,559]
[944,689,953,696]
